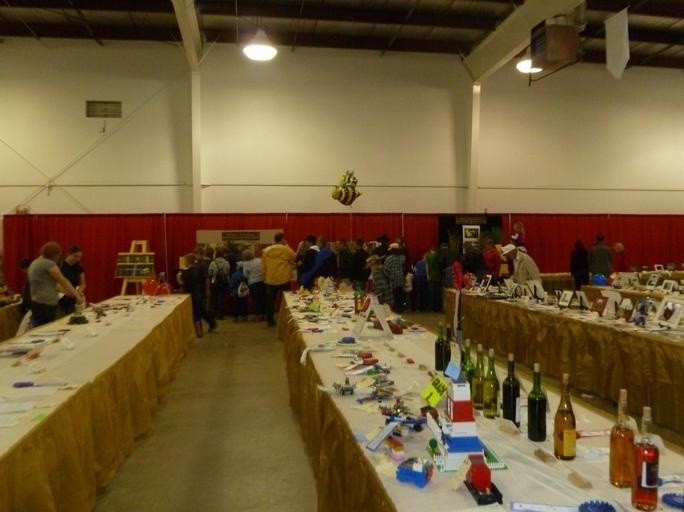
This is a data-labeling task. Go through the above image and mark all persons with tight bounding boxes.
[56,245,86,314]
[412,236,543,311]
[176,232,408,337]
[567,237,589,290]
[19,256,34,314]
[610,241,631,274]
[585,230,611,279]
[24,240,83,327]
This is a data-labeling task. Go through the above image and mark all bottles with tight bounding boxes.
[434,320,579,461]
[608,384,660,511]
[354,282,365,312]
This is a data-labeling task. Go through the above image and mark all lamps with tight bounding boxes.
[515,45,544,75]
[242,28,279,63]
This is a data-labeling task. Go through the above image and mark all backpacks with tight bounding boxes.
[213,259,229,297]
[402,272,415,293]
[237,281,250,298]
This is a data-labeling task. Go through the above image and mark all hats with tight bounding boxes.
[501,243,516,256]
[387,242,401,251]
